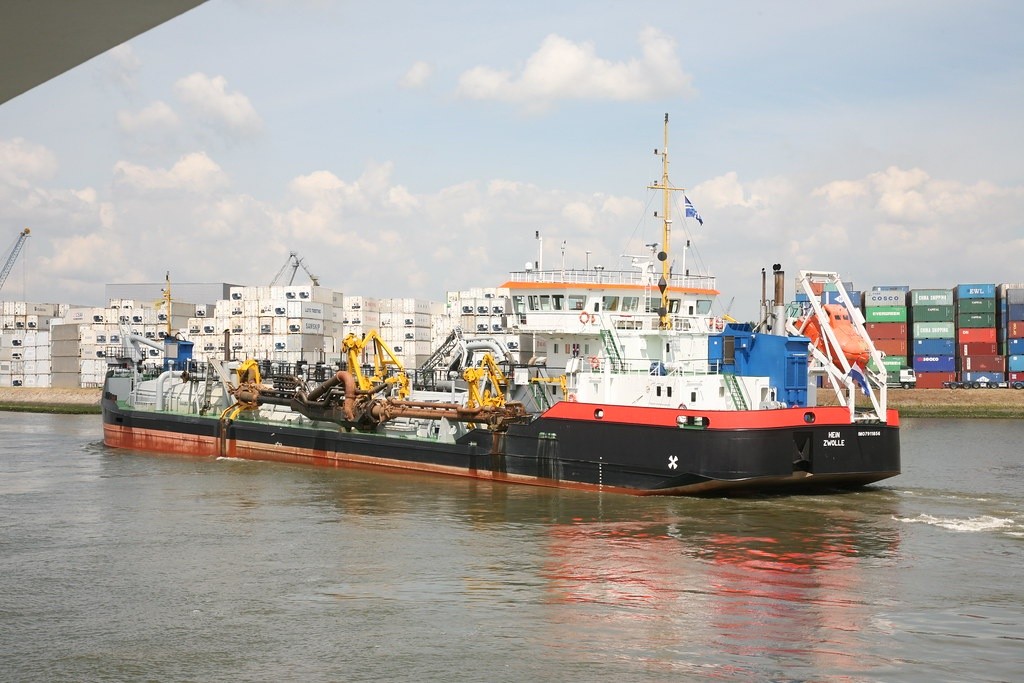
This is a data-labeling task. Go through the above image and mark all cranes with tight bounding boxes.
[0,226,34,290]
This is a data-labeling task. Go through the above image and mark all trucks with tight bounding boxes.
[865,363,917,389]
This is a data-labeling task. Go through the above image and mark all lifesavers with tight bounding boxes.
[709,317,723,329]
[568,393,577,402]
[678,404,687,409]
[579,311,590,324]
[590,356,600,368]
[792,404,799,408]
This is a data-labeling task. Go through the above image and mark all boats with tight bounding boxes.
[95,110,904,497]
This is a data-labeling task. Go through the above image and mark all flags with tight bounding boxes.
[685,196,703,226]
